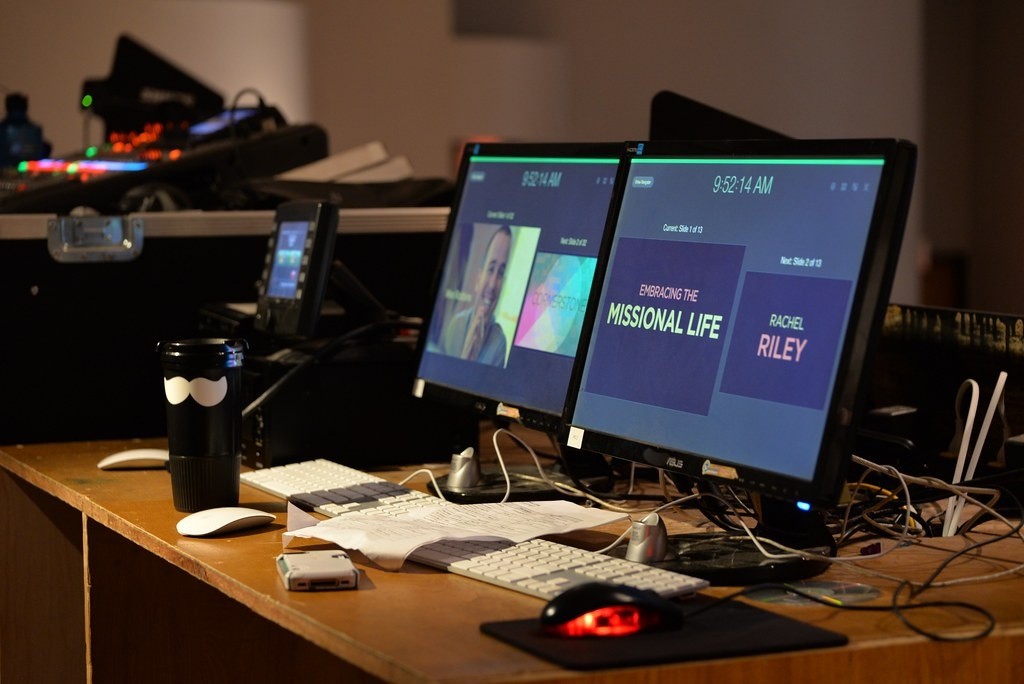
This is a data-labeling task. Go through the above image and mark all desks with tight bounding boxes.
[0,420,1024,684]
[0,207,450,239]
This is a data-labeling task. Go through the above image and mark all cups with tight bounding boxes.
[159,338,245,512]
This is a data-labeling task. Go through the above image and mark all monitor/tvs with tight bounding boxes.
[255,198,341,338]
[415,136,917,593]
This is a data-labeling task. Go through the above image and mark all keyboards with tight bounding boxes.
[238,458,456,519]
[405,537,709,600]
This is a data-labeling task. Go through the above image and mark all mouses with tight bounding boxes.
[176,506,276,537]
[538,580,684,635]
[96,448,170,470]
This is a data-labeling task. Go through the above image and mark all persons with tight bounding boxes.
[436,224,514,369]
